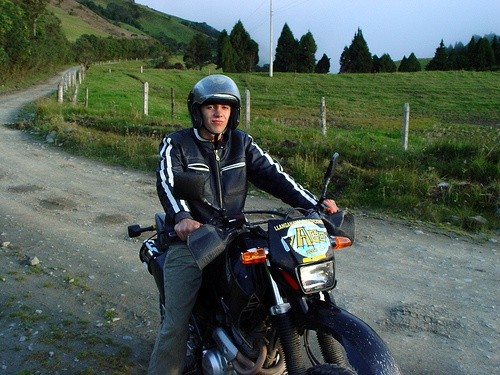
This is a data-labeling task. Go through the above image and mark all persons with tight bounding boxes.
[145,73,339,375]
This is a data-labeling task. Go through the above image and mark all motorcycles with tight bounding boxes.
[127,153,404,375]
